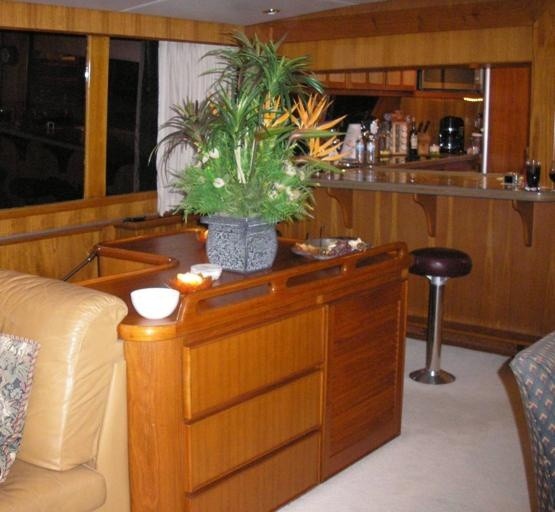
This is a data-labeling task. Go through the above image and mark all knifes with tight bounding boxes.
[417,120,431,137]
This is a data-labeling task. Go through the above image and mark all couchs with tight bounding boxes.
[0,269,131,512]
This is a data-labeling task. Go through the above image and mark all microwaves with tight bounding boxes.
[419,65,485,95]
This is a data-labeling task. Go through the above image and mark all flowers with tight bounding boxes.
[147,26,350,227]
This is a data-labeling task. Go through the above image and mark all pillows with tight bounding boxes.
[0,335,40,484]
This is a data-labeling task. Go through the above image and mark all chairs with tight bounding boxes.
[497,329,555,512]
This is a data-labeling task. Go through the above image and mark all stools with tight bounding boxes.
[409,247,472,384]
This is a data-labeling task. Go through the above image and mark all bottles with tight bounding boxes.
[408,116,418,156]
[366,134,376,164]
[355,134,365,163]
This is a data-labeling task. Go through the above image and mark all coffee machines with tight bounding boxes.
[440,115,466,155]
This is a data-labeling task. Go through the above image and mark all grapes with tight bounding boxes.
[328,239,352,257]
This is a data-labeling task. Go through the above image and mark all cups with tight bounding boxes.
[524,160,541,191]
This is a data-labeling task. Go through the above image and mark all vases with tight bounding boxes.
[199,214,285,274]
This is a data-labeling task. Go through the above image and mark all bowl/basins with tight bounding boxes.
[129,287,180,321]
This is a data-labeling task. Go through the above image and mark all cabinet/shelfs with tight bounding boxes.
[307,69,417,97]
